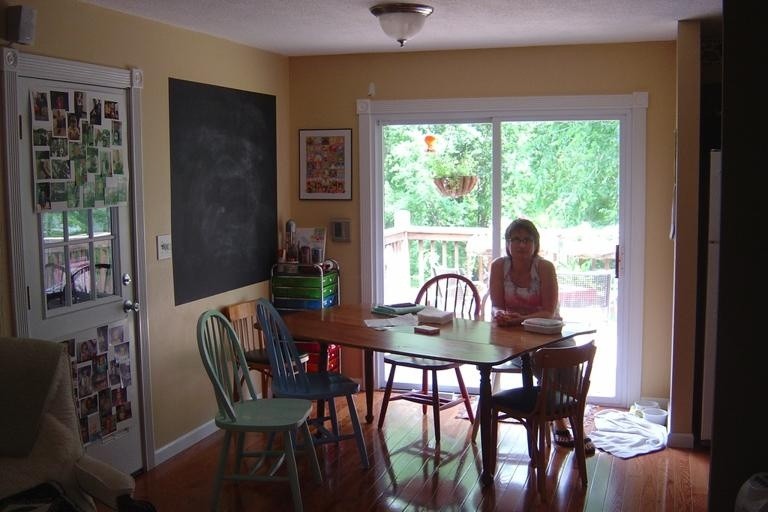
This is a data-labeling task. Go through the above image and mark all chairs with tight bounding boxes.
[195,296,371,512]
[1,337,157,510]
[378,274,481,443]
[482,340,595,493]
[470,286,528,442]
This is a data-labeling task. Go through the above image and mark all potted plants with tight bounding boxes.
[432,156,479,196]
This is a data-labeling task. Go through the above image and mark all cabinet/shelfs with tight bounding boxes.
[269,258,341,374]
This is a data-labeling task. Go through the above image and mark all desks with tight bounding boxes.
[254,303,597,489]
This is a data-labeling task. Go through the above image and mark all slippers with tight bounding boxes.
[550,422,575,448]
[583,435,596,455]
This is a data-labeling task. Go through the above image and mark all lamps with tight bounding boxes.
[369,3,435,48]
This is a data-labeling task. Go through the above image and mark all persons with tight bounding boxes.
[488,217,595,455]
[31,89,128,211]
[55,324,132,444]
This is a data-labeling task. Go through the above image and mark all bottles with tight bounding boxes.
[286,220,296,254]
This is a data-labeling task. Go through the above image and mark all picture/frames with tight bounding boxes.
[298,128,353,201]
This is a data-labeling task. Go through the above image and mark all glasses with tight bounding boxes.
[507,238,536,245]
